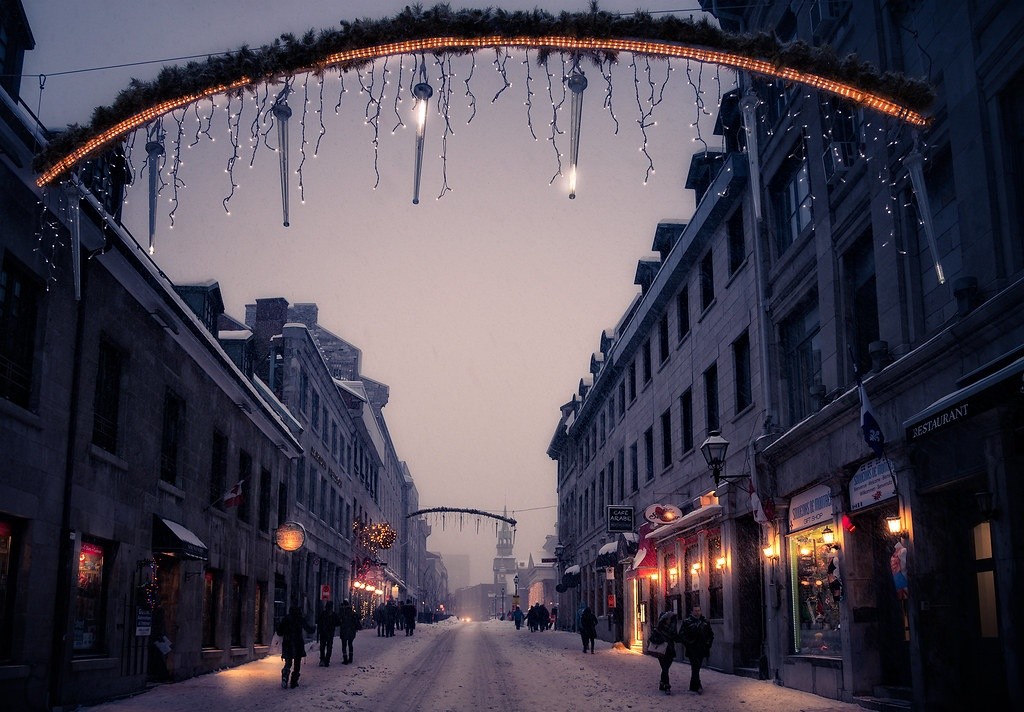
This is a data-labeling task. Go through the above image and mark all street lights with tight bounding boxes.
[501,586,504,621]
[513,575,519,595]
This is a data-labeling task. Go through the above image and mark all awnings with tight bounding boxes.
[595,551,619,567]
[562,573,581,586]
[162,518,210,551]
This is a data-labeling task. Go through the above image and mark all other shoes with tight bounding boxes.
[658,681,672,695]
[319,660,331,667]
[689,685,704,693]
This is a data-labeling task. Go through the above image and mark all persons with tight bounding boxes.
[375,599,417,638]
[649,604,715,694]
[578,607,598,654]
[827,544,840,602]
[276,604,316,689]
[512,607,525,630]
[314,598,359,667]
[890,542,908,600]
[524,603,557,632]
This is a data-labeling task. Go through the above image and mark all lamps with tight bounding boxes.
[738,69,767,225]
[669,568,680,584]
[951,275,981,315]
[975,486,1000,522]
[60,167,87,305]
[236,402,253,415]
[866,340,891,372]
[898,122,947,289]
[410,54,435,204]
[267,78,296,229]
[555,538,576,563]
[145,307,179,335]
[693,560,703,579]
[885,514,909,539]
[562,49,588,201]
[810,384,828,413]
[762,539,778,559]
[716,554,726,574]
[276,444,288,452]
[699,428,752,494]
[820,523,841,550]
[144,118,170,253]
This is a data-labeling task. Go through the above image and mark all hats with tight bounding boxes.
[325,601,333,609]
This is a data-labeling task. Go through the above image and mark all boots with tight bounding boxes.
[349,652,354,664]
[290,670,300,689]
[281,668,291,688]
[342,653,348,665]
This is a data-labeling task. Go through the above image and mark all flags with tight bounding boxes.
[852,363,889,459]
[749,478,771,525]
[220,477,250,502]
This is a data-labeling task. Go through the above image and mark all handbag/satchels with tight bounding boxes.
[267,632,284,655]
[352,612,362,630]
[648,641,669,656]
